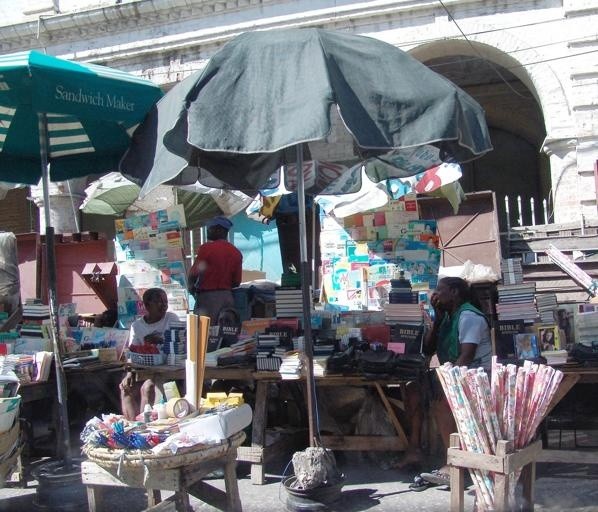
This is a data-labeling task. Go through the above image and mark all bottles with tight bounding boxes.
[142,399,167,425]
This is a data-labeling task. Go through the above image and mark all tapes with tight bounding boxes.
[165,398,189,418]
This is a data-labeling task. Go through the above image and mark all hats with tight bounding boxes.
[209,216,234,230]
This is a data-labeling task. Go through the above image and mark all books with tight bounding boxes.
[199,268,428,384]
[13,296,49,335]
[496,279,558,324]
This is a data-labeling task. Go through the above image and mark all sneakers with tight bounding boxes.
[420,468,450,485]
[409,474,429,491]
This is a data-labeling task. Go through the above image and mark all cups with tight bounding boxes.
[369,342,384,351]
[66,311,79,327]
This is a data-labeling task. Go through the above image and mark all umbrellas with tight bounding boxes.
[121,23,495,437]
[0,42,166,461]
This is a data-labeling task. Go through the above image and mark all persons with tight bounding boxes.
[540,329,555,351]
[181,214,243,321]
[517,334,537,360]
[114,287,179,422]
[379,275,493,471]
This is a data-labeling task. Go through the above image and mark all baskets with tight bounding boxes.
[128,351,166,366]
